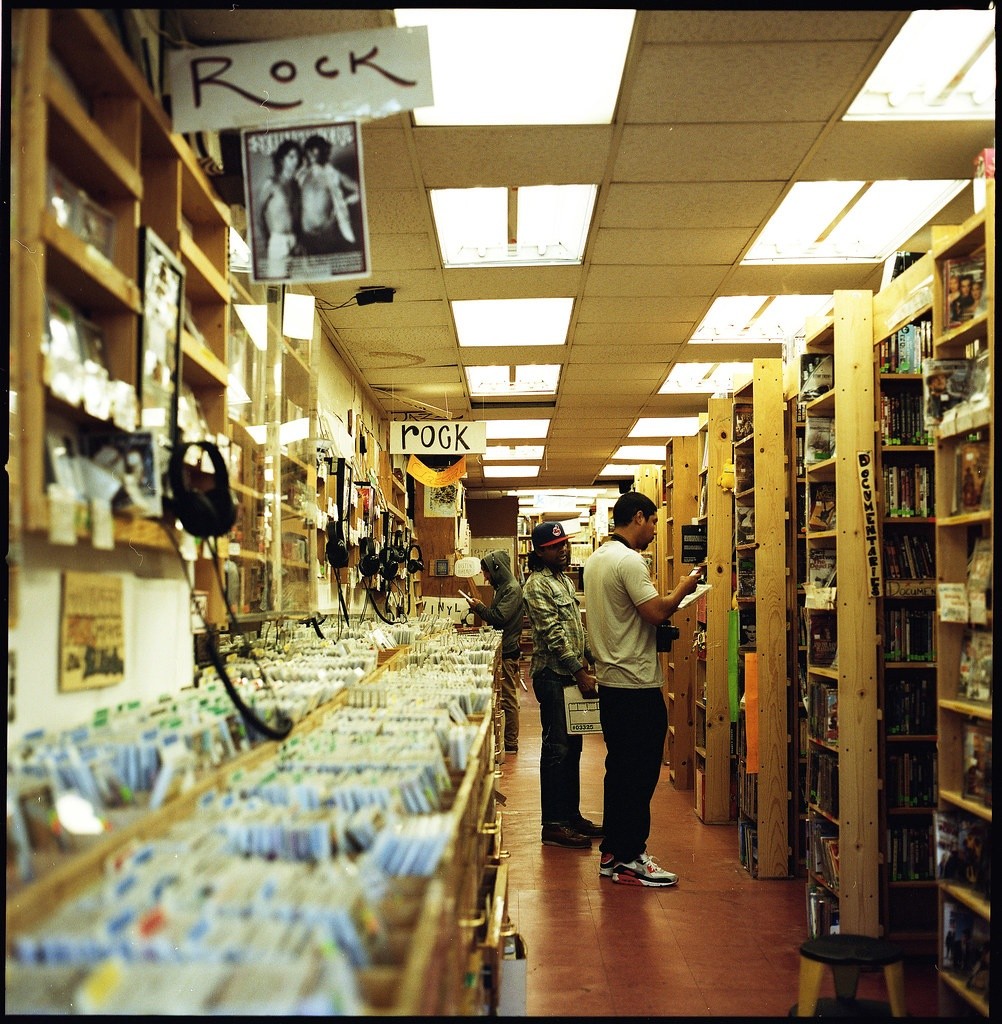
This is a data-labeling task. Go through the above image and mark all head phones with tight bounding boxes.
[492,553,500,571]
[326,520,349,569]
[169,440,236,537]
[393,530,407,563]
[379,547,398,580]
[406,545,425,573]
[359,537,380,577]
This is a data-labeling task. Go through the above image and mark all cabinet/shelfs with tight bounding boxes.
[732,357,789,878]
[785,334,805,878]
[693,397,740,826]
[873,250,936,956]
[932,178,993,1023]
[6,8,522,1015]
[516,467,667,767]
[806,289,878,938]
[667,436,696,790]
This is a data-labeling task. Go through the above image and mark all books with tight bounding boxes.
[691,255,992,1012]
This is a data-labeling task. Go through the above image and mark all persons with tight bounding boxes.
[252,130,364,281]
[583,492,704,887]
[466,550,525,750]
[521,521,607,849]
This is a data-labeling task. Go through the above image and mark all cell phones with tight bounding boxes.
[688,566,702,577]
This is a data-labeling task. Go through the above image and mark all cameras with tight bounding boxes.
[656,625,680,640]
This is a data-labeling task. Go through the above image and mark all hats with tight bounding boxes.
[532,522,575,547]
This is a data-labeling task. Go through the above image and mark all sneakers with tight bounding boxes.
[578,816,603,837]
[504,745,518,754]
[541,824,592,848]
[600,853,679,887]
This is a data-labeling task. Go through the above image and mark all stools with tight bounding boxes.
[798,934,909,1017]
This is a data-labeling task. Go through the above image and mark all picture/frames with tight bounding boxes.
[134,224,188,433]
